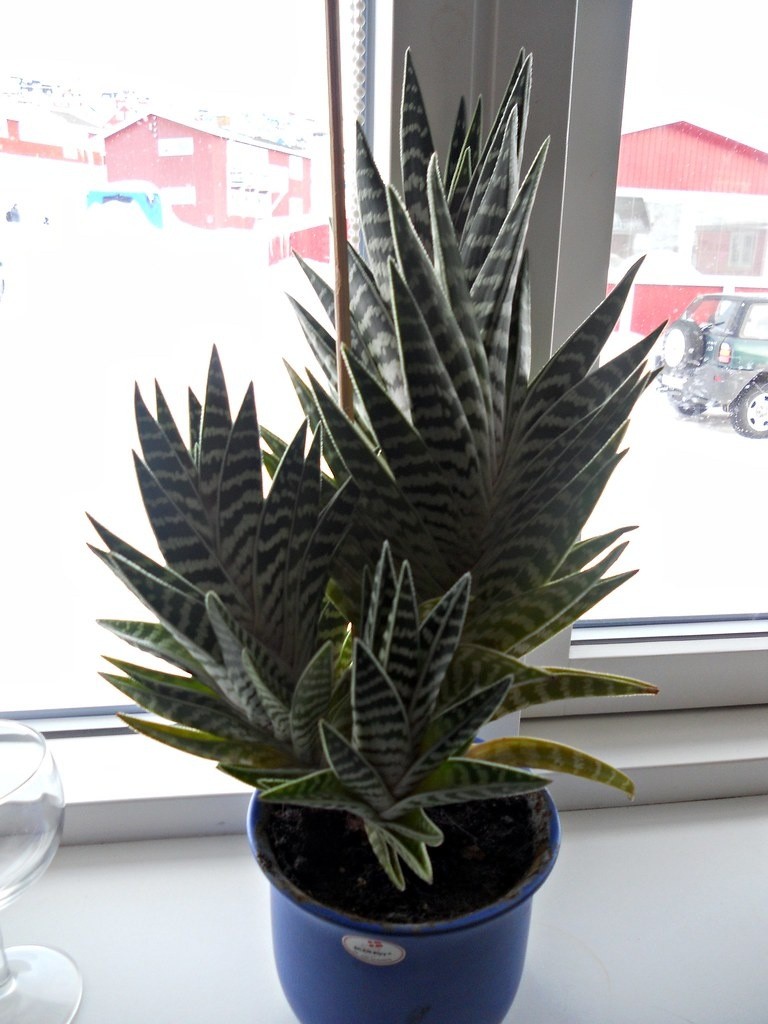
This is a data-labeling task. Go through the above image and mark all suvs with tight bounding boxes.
[656,290,768,439]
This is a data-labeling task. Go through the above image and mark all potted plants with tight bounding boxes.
[81,40,674,1024]
